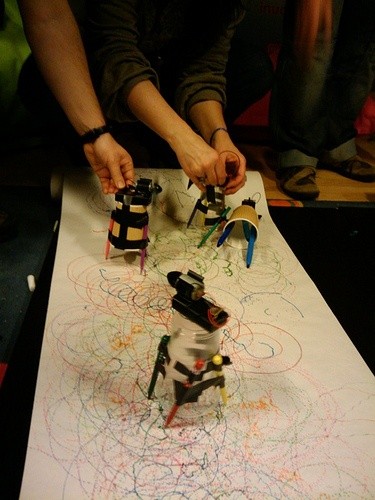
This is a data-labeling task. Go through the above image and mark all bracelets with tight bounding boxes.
[210,127,228,146]
[80,125,109,145]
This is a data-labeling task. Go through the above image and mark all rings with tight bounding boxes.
[199,177,206,184]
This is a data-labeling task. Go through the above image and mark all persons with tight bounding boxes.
[0,0,375,243]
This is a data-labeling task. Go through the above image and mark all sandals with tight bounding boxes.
[280,166,320,201]
[320,156,375,182]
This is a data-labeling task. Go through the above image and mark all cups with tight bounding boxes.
[224,204,260,250]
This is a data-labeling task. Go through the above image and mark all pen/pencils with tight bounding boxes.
[213,355,227,406]
[187,199,201,229]
[148,335,169,399]
[217,221,235,247]
[198,207,230,248]
[105,219,114,260]
[164,360,204,427]
[140,225,148,272]
[187,179,193,190]
[244,222,256,268]
